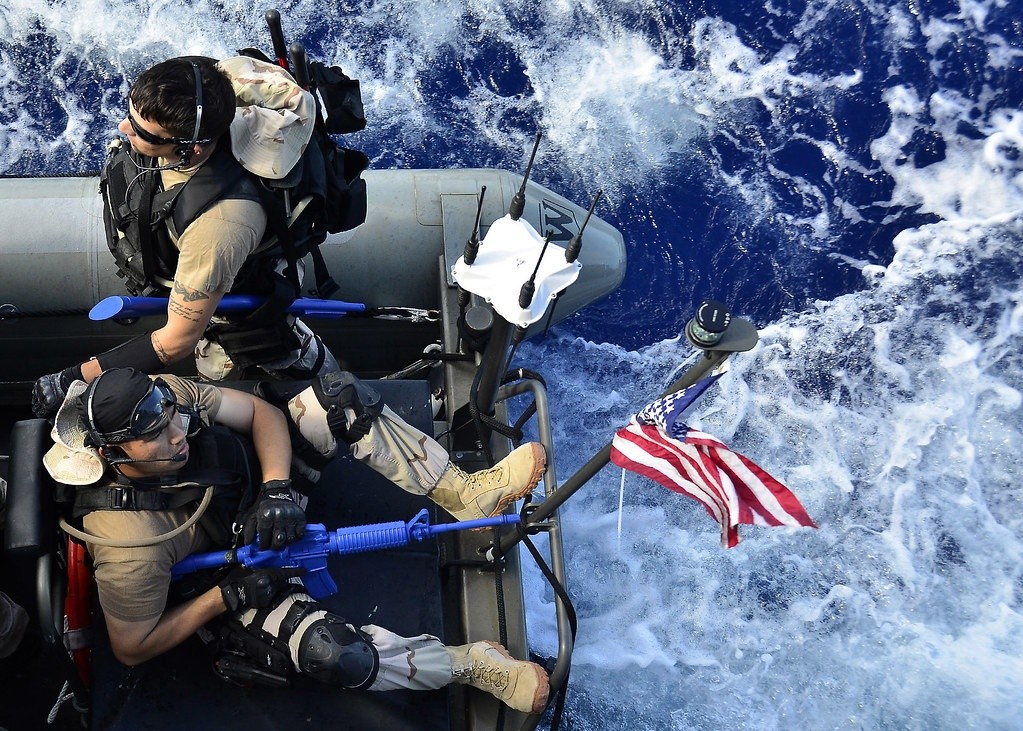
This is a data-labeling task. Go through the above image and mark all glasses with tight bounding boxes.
[97,376,177,443]
[127,91,182,146]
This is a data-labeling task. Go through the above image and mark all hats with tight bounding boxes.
[212,54,317,180]
[41,378,107,486]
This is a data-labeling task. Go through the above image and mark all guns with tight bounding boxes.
[170,510,520,603]
[89,293,416,329]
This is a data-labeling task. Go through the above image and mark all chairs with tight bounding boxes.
[6,376,460,731]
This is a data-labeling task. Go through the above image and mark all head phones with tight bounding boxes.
[170,55,203,164]
[87,368,125,466]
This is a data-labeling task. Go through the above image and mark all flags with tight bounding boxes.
[607,369,821,550]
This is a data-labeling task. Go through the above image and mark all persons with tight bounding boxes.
[46,363,556,718]
[27,51,350,418]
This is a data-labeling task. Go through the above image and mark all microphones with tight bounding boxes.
[123,141,190,171]
[110,457,187,463]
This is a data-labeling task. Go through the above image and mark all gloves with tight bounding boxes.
[31,368,76,419]
[217,566,308,614]
[243,478,306,551]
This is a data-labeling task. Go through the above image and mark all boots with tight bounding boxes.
[426,441,548,533]
[446,638,551,716]
